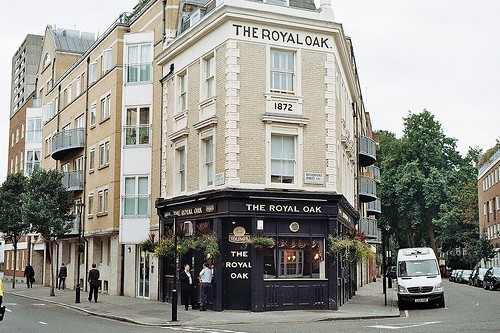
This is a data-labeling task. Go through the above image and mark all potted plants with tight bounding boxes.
[244,238,274,250]
[140,235,159,259]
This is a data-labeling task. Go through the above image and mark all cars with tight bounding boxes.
[386,266,397,279]
[449,268,489,287]
[483,267,500,291]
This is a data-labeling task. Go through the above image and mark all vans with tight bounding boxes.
[397,247,445,310]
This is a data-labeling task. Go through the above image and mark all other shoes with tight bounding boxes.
[192,306,198,310]
[199,308,207,312]
[30,285,33,289]
[27,286,30,288]
[87,298,91,302]
[184,306,189,311]
[94,300,97,303]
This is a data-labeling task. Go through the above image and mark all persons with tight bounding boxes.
[88,263,100,303]
[198,263,212,310]
[24,262,35,288]
[180,264,197,310]
[57,263,67,291]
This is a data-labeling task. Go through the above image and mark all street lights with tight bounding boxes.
[170,212,180,322]
[385,221,393,289]
[75,196,85,303]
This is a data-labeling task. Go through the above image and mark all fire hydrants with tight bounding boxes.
[388,272,392,288]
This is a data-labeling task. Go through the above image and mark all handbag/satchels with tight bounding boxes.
[31,277,36,283]
